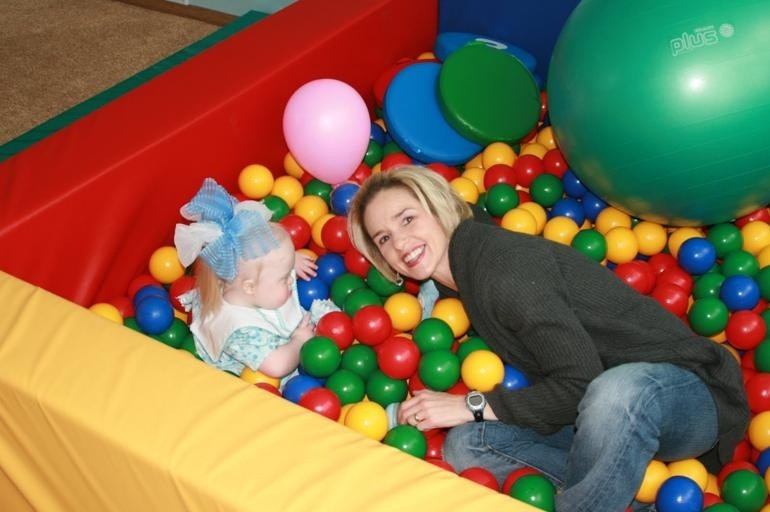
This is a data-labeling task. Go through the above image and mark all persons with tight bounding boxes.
[172,178,341,396]
[347,165,750,512]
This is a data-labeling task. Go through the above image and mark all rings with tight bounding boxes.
[415,415,420,422]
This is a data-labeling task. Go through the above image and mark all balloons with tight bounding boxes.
[283,78,371,190]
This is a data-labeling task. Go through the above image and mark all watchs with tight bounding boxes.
[465,389,486,422]
